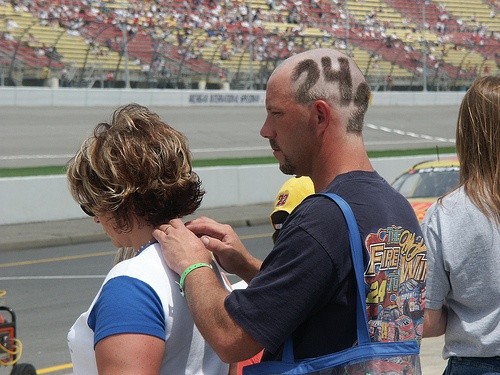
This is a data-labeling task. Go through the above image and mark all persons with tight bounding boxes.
[419,75,500,375]
[0,0,500,90]
[152,47,428,375]
[66,103,235,375]
[270,172,315,244]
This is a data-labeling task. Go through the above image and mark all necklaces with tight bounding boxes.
[133,238,158,255]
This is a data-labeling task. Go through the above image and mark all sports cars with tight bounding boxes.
[389,160,466,224]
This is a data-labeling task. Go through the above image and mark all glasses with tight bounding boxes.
[80,203,95,217]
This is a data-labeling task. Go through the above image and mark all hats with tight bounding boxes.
[269,175,316,230]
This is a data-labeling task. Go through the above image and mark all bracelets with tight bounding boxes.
[174,263,214,298]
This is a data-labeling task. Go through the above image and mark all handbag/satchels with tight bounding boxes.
[242,192,422,375]
